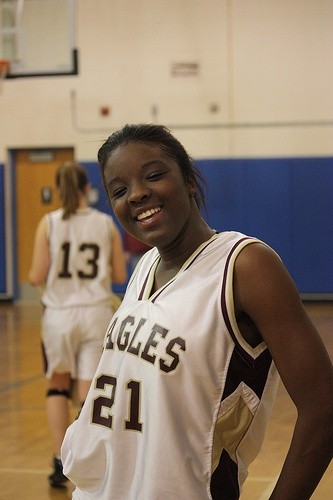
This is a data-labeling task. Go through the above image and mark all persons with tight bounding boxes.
[29,160,126,488]
[56,125,332,500]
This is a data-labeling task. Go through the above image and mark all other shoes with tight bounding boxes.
[48,473,69,485]
